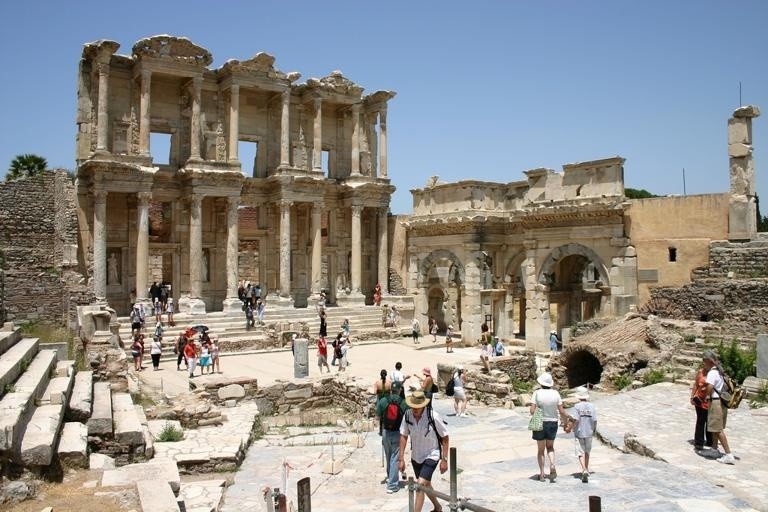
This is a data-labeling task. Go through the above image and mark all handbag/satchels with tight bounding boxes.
[527,407,544,433]
[432,383,439,393]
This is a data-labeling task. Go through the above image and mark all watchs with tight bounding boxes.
[442,456,447,462]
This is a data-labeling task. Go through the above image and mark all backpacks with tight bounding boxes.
[446,373,460,397]
[382,395,407,432]
[709,367,746,410]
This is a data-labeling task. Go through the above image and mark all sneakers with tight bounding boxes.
[387,485,401,494]
[581,470,589,483]
[716,453,735,465]
[698,447,719,458]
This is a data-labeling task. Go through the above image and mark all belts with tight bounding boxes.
[711,397,720,401]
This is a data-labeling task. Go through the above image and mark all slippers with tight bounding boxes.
[550,465,557,481]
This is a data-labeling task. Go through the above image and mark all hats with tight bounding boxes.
[405,390,431,410]
[536,372,554,388]
[422,367,431,376]
[576,385,590,400]
[155,297,159,303]
[448,324,454,329]
[390,381,400,392]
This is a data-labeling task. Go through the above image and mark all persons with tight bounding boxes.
[411,319,419,344]
[381,304,388,326]
[291,290,353,375]
[429,319,438,342]
[689,368,712,450]
[567,385,596,483]
[493,337,504,356]
[529,373,565,482]
[201,250,208,282]
[697,351,734,464]
[372,284,381,307]
[128,281,223,377]
[549,330,561,352]
[444,325,453,353]
[106,252,118,284]
[397,391,449,512]
[237,280,265,329]
[375,361,469,493]
[391,306,400,337]
[477,324,492,376]
